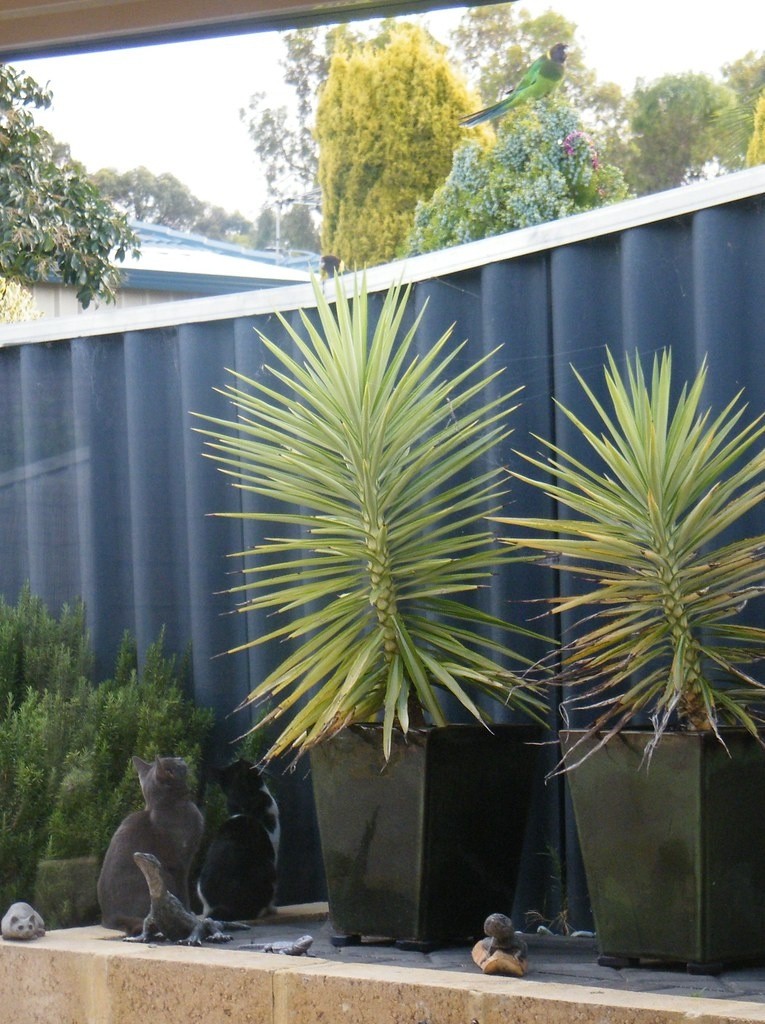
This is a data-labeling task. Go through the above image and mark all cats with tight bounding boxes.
[2,901,47,940]
[93,753,205,933]
[198,757,280,921]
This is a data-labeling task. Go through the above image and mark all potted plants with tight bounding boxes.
[188,263,556,952]
[482,343,765,977]
